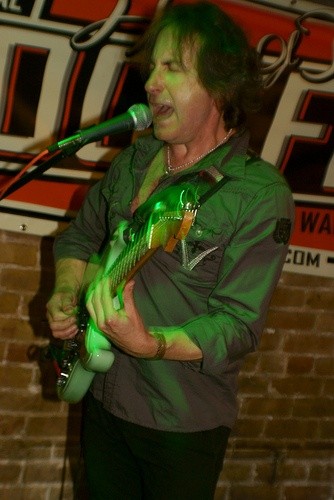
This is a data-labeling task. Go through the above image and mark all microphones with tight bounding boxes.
[48,104,152,153]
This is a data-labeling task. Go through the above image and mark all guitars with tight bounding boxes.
[41,203,200,403]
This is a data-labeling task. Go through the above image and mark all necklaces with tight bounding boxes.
[166,127,238,171]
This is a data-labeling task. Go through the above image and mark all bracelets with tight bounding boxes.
[152,330,168,365]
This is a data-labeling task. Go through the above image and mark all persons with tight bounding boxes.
[47,3,296,494]
